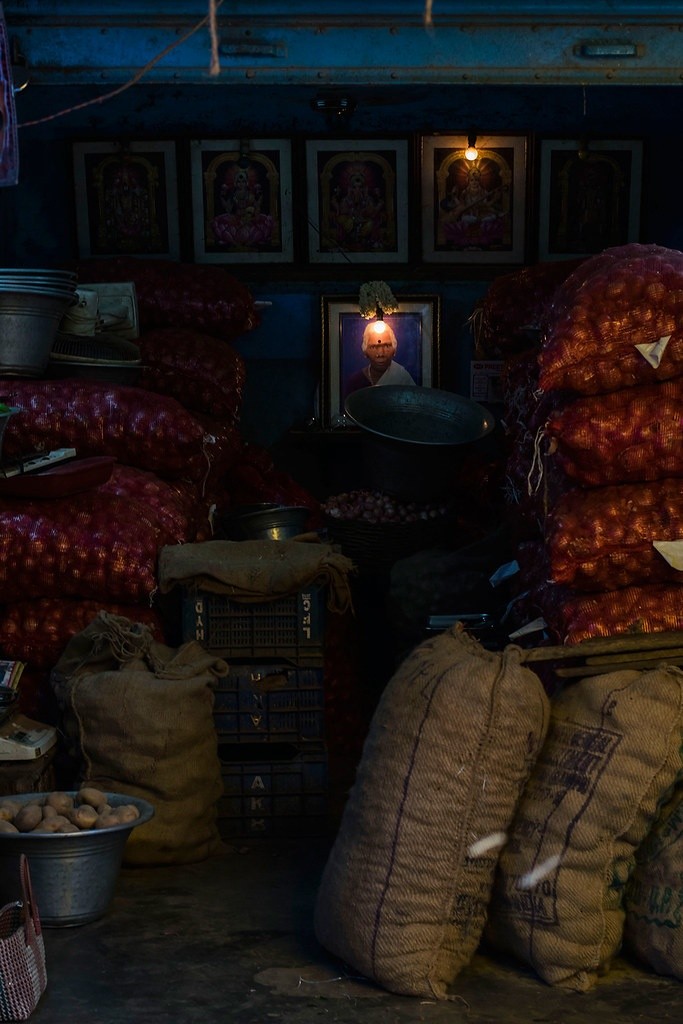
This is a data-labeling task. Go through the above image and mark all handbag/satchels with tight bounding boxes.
[0,853,48,1021]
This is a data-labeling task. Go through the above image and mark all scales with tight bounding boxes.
[0,706,58,760]
[405,592,500,655]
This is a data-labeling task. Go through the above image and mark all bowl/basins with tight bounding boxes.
[234,506,311,542]
[342,385,495,468]
[0,268,80,378]
[0,791,157,929]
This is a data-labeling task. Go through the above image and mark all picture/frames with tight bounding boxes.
[62,128,651,282]
[320,293,441,430]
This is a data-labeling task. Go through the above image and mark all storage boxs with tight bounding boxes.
[182,583,329,847]
[0,743,58,796]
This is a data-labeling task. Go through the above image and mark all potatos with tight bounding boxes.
[0,241,683,835]
